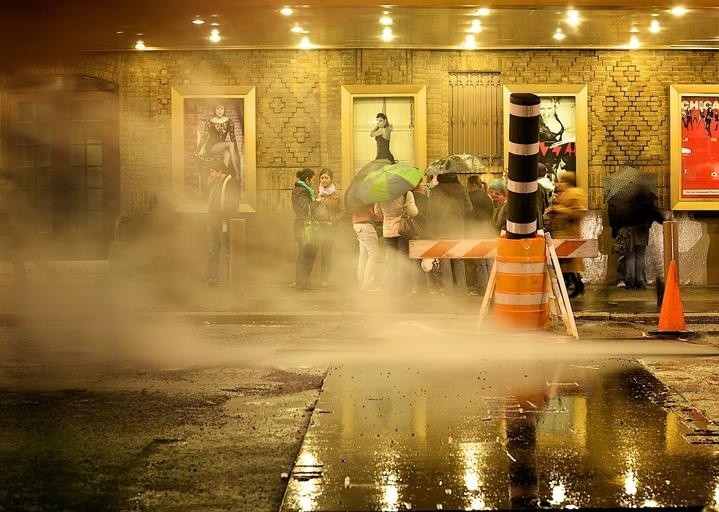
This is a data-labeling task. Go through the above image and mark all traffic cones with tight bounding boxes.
[648,260,695,335]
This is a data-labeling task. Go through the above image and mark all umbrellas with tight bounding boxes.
[423,153,486,175]
[344,157,391,213]
[604,164,659,216]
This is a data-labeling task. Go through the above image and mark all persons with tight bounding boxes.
[370,113,394,164]
[681,103,719,137]
[205,158,240,283]
[287,168,347,287]
[292,169,323,290]
[352,163,588,298]
[191,101,240,181]
[608,195,665,290]
[538,96,576,186]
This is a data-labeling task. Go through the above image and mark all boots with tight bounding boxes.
[570,282,584,298]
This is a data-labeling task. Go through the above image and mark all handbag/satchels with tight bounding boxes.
[615,227,633,259]
[301,217,326,248]
[398,215,422,236]
[421,258,440,272]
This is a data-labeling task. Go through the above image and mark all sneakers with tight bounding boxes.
[616,280,648,290]
[358,284,377,292]
[411,287,482,296]
[295,281,328,290]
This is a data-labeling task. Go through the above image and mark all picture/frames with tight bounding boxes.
[171,84,257,215]
[669,84,719,211]
[502,83,589,207]
[340,84,427,200]
